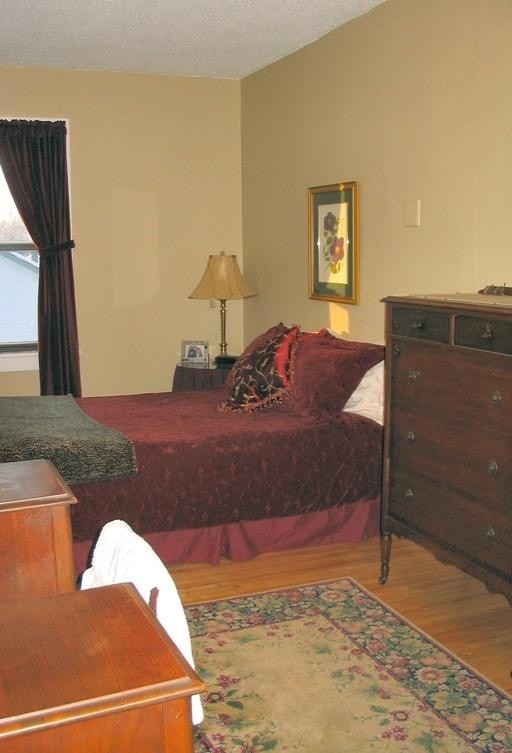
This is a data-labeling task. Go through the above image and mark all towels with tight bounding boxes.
[81,519,205,730]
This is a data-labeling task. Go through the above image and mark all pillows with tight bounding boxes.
[224,320,285,389]
[215,323,304,414]
[282,327,385,422]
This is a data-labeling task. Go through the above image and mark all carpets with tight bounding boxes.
[178,573,512,752]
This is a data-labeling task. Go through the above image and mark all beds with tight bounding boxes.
[0,324,388,580]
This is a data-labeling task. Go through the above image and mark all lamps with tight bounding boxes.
[188,248,259,354]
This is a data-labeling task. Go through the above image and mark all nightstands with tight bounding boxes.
[171,355,241,394]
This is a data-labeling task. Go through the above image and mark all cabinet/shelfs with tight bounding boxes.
[0,579,210,752]
[374,288,511,607]
[0,456,80,603]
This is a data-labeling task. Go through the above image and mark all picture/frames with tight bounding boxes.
[181,338,211,364]
[306,177,360,305]
